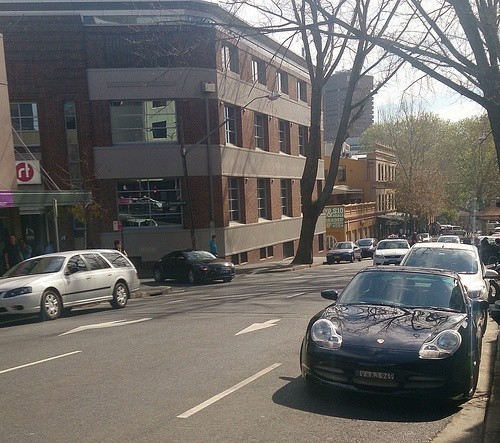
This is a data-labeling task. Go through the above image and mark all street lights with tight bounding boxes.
[176,92,282,250]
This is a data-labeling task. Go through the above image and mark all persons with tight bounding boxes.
[462,223,478,237]
[411,231,417,245]
[209,235,218,256]
[3,234,31,272]
[113,240,128,257]
[474,238,500,265]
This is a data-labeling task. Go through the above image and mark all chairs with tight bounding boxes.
[426,282,459,309]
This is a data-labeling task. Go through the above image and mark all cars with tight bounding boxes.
[300,265,490,404]
[435,235,462,243]
[136,196,164,208]
[1,249,140,318]
[153,250,237,286]
[355,238,378,258]
[325,241,362,262]
[372,233,412,267]
[416,232,433,243]
[398,242,498,302]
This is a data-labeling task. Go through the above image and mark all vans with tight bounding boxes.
[443,225,467,236]
[126,218,159,227]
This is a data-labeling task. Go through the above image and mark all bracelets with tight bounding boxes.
[5,264,9,266]
